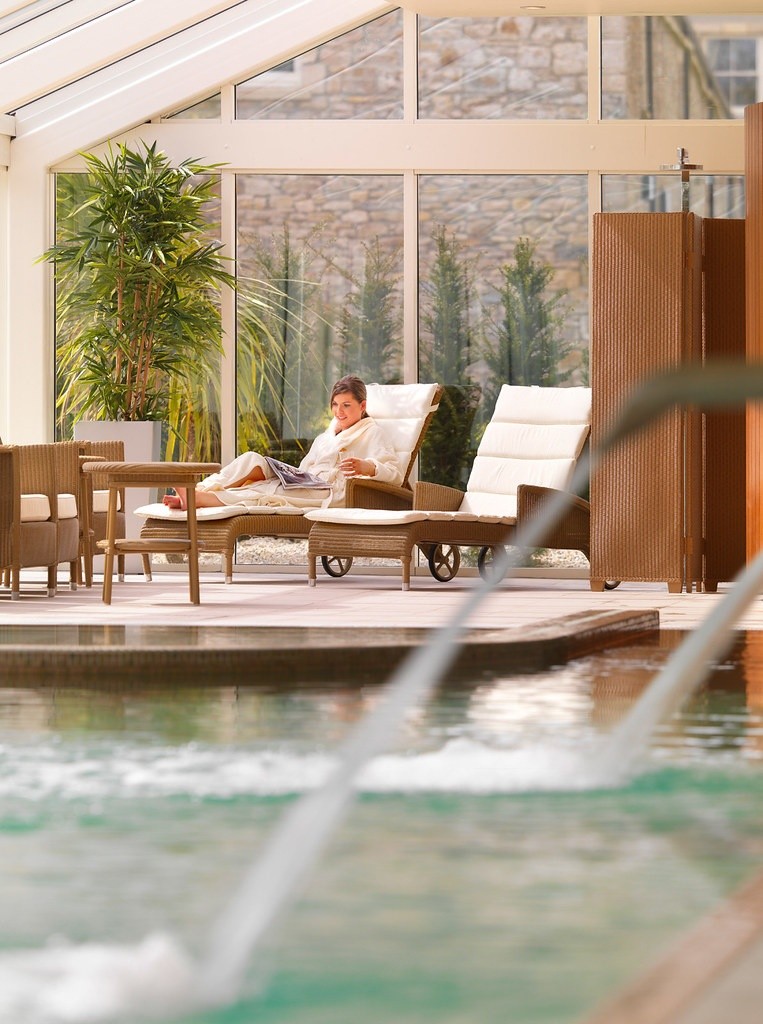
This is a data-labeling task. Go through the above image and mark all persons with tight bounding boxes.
[162,375,404,510]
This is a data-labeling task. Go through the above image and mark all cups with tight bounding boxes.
[339,451,354,473]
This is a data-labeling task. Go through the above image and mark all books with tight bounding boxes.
[264,456,332,489]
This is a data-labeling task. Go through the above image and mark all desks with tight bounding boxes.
[82,461,223,606]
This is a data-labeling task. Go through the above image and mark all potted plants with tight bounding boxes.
[32,137,253,577]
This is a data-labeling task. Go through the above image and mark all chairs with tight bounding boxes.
[131,380,461,585]
[303,384,621,592]
[0,440,126,601]
